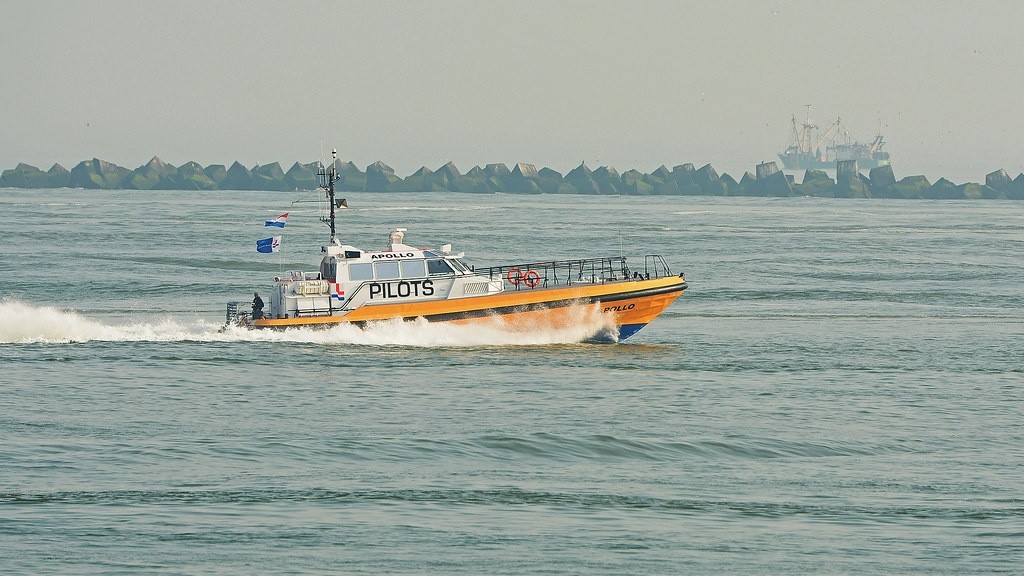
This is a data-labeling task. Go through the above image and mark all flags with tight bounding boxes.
[256,236,281,253]
[264,212,289,228]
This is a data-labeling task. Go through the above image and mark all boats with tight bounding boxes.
[776,104,892,169]
[218,141,689,344]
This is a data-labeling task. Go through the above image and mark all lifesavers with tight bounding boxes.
[524,270,541,288]
[507,267,525,285]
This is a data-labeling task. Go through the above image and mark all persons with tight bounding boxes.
[252,292,265,319]
[316,273,324,280]
